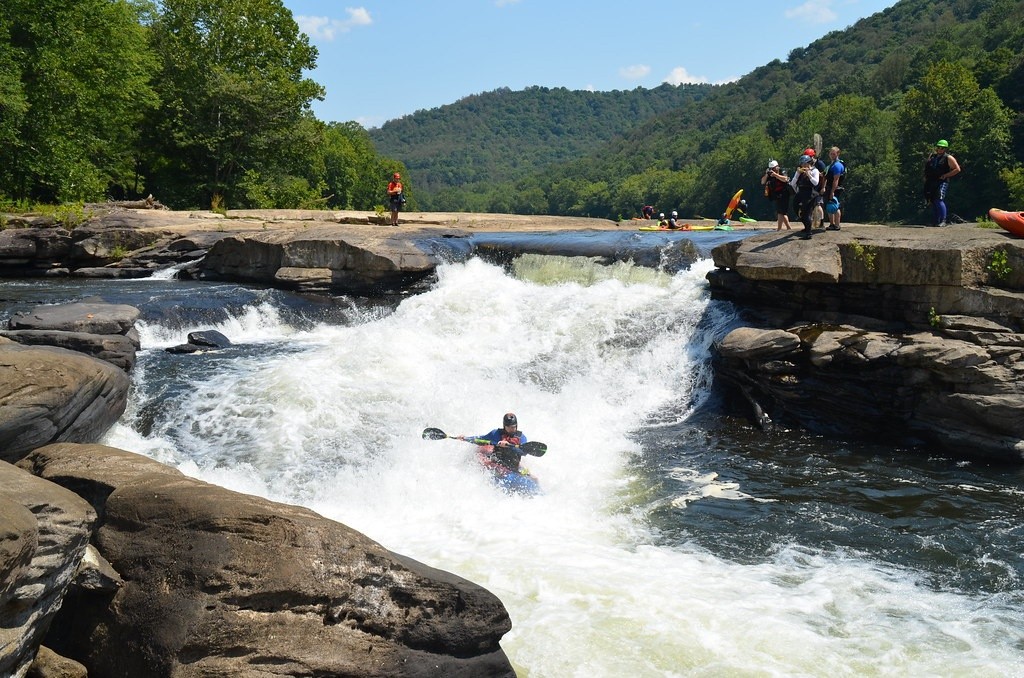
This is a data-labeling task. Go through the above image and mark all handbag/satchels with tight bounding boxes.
[765,181,769,196]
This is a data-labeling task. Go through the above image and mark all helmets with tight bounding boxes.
[672,211,678,216]
[803,148,814,157]
[826,196,840,214]
[722,213,727,217]
[937,140,948,148]
[741,200,746,204]
[660,213,665,217]
[503,413,517,427]
[799,155,811,165]
[769,161,779,168]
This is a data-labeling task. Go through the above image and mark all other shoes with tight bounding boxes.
[826,224,840,230]
[799,235,811,240]
[392,223,398,226]
[935,222,947,227]
[810,228,824,234]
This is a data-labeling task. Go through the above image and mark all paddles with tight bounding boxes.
[736,207,751,218]
[677,223,690,230]
[810,132,825,232]
[422,427,548,458]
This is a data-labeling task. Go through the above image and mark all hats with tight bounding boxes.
[394,173,400,179]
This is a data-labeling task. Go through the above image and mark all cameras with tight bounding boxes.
[767,168,773,176]
[801,168,806,172]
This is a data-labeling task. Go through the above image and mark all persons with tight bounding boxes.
[925,139,961,227]
[761,146,846,240]
[717,212,730,226]
[740,199,747,218]
[458,413,527,472]
[386,173,402,227]
[659,211,688,230]
[641,205,657,219]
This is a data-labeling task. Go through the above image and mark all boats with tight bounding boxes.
[639,224,714,232]
[477,444,541,494]
[715,224,734,231]
[632,217,647,220]
[740,217,757,223]
[725,189,743,220]
